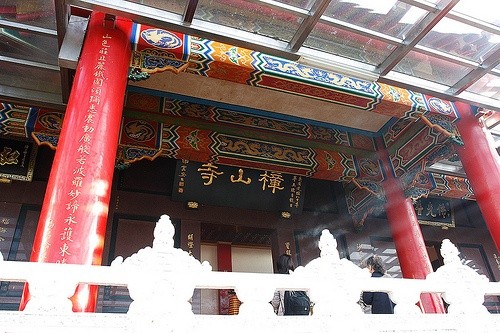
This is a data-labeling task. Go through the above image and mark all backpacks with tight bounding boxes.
[283,290,311,316]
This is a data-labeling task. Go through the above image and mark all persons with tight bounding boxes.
[363,256,395,314]
[271,254,313,315]
